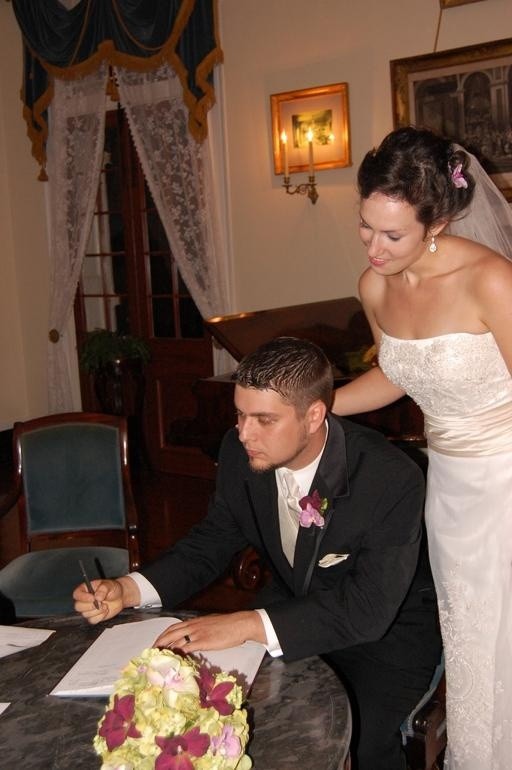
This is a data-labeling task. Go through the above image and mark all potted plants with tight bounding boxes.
[80,329,150,408]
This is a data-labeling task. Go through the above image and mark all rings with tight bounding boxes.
[184,635,191,643]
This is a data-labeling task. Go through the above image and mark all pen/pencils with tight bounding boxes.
[78,559,99,610]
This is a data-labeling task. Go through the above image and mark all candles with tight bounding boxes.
[283,129,290,184]
[306,128,315,181]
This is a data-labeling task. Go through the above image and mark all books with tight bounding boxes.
[51,616,267,705]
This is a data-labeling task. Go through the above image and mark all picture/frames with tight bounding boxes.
[268,82,355,172]
[385,35,510,201]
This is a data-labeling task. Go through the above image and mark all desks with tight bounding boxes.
[0,611,360,770]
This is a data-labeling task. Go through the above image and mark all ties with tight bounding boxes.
[284,473,303,530]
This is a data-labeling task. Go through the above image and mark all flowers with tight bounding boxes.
[91,643,254,770]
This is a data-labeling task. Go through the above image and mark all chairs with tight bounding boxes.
[1,410,152,621]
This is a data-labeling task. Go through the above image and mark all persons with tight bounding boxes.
[235,127,512,770]
[73,336,442,770]
